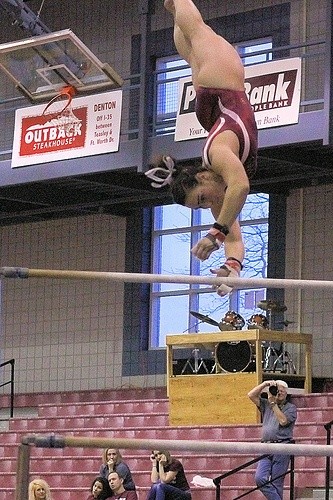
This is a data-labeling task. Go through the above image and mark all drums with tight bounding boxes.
[215,340,252,373]
[248,314,267,330]
[218,310,245,331]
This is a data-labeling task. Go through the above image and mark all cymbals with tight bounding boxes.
[257,300,287,311]
[278,321,297,324]
[190,311,219,326]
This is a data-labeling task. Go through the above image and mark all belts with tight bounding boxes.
[262,440,282,442]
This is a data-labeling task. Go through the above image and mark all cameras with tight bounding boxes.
[269,386,277,396]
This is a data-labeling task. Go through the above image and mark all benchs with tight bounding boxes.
[0,385,333,500]
